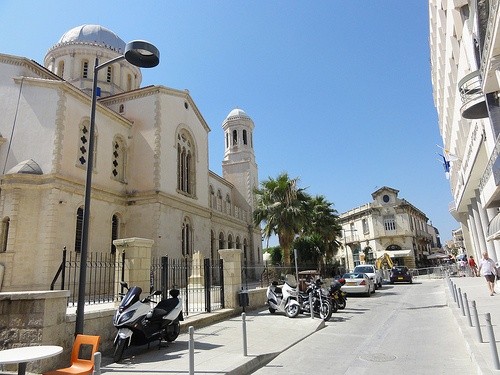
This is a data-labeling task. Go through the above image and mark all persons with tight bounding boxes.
[448,259,455,265]
[468,255,478,277]
[478,252,497,296]
[458,255,468,270]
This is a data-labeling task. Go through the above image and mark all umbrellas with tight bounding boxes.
[427,252,449,261]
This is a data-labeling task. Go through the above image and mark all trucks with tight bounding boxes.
[353,264,383,290]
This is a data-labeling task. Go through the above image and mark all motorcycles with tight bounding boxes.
[297,273,349,321]
[112,280,184,362]
[265,274,302,318]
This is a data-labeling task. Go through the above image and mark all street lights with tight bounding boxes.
[74,39,162,358]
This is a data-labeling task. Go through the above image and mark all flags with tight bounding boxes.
[440,149,451,179]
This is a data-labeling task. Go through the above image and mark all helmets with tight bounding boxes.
[338,278,345,284]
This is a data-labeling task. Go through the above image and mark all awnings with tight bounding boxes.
[377,250,412,258]
[487,213,500,241]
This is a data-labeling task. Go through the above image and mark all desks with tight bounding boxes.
[0,346,63,375]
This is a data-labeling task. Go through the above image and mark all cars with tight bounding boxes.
[388,266,413,285]
[340,272,377,297]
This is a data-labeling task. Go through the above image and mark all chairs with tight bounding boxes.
[43,334,101,375]
